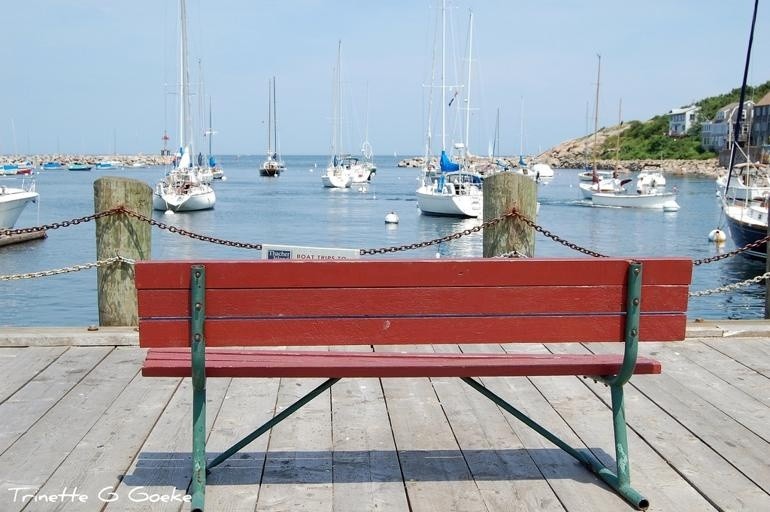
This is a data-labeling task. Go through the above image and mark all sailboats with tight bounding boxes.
[322,39,378,189]
[415,1,540,218]
[715,1,770,263]
[578,52,680,213]
[273,75,288,172]
[259,79,281,178]
[152,1,224,217]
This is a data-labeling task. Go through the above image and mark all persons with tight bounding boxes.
[636,177,644,195]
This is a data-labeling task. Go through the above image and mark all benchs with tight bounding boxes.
[134,256,693,512]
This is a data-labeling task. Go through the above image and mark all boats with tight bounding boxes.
[714,162,770,202]
[0,121,146,175]
[0,176,40,232]
[527,154,554,178]
